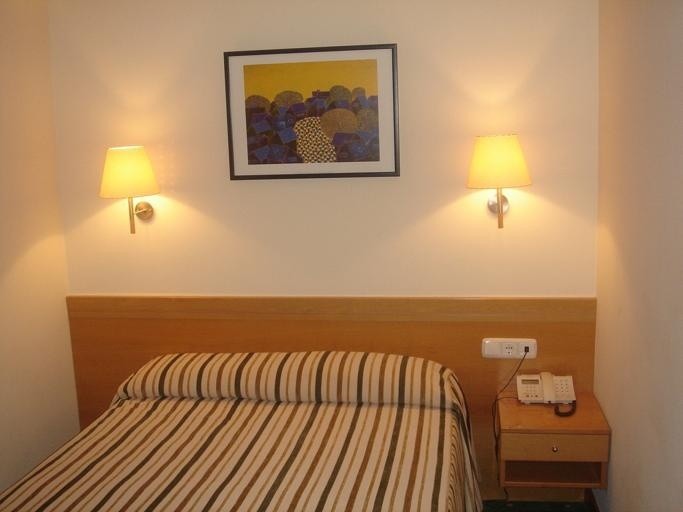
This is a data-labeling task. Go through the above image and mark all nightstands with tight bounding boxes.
[487,388,614,492]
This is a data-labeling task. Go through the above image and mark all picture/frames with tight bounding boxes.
[218,38,406,183]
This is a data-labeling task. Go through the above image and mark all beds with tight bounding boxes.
[0,342,490,510]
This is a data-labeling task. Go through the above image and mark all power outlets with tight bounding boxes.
[480,336,538,360]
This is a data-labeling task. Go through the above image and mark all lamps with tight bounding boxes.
[95,139,162,241]
[462,126,533,230]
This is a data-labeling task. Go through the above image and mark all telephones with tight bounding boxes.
[516,371,577,405]
